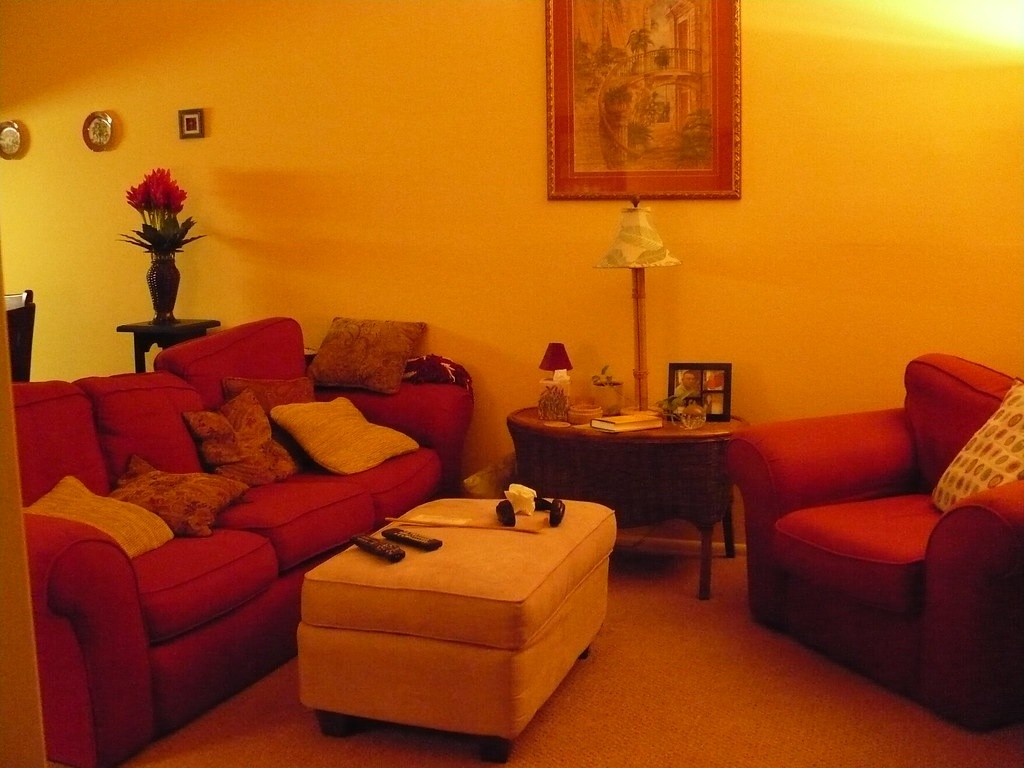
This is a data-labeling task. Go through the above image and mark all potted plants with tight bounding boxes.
[591,363,694,415]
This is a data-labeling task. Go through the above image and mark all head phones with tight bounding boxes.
[496,496,565,528]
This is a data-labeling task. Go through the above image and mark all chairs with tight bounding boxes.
[4,290,36,382]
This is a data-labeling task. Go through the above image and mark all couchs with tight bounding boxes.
[13,316,473,768]
[725,354,1023,733]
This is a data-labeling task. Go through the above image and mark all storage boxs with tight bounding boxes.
[539,378,573,420]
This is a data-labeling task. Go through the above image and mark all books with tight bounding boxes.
[590,414,662,432]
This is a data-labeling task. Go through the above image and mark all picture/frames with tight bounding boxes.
[669,362,731,422]
[177,109,204,139]
[544,0,742,200]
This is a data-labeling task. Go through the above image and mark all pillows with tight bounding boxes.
[270,397,420,476]
[463,451,517,500]
[106,455,251,536]
[932,379,1024,513]
[305,317,427,393]
[222,377,314,442]
[22,476,174,559]
[182,386,303,487]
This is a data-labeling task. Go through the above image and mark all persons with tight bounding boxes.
[674,371,697,398]
[704,394,713,414]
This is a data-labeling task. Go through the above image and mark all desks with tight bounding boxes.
[116,320,221,373]
[507,406,749,601]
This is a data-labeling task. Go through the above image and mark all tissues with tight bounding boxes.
[537,369,571,421]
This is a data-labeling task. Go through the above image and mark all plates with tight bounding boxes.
[0,121,22,160]
[82,111,114,152]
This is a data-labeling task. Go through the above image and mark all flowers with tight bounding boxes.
[115,169,207,252]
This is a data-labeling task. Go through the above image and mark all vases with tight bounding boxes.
[146,252,180,324]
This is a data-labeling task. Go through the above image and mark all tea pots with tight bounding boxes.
[671,402,707,429]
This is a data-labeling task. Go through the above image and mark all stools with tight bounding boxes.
[296,497,618,763]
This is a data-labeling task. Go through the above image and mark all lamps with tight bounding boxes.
[539,343,573,374]
[591,195,682,411]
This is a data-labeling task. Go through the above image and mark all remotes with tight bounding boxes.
[349,534,406,563]
[382,528,442,552]
